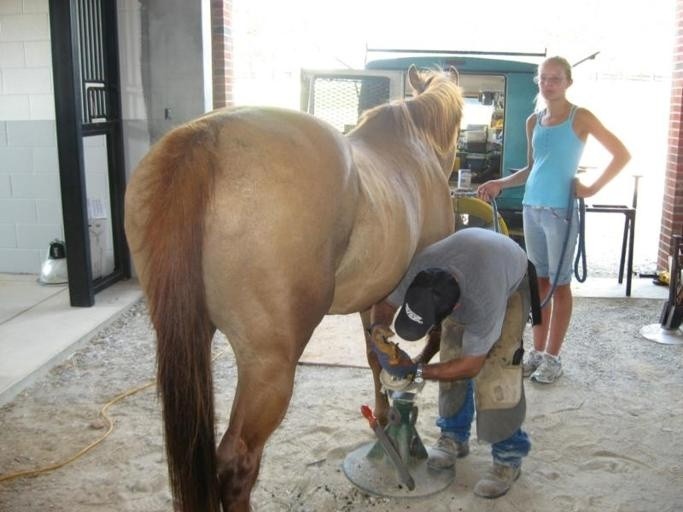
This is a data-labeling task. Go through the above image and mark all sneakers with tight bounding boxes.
[474,463,520,498]
[530,354,562,383]
[427,436,469,471]
[523,351,542,377]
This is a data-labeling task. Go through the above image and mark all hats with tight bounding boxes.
[394,268,459,341]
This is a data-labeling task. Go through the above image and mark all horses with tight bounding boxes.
[123,64,464,512]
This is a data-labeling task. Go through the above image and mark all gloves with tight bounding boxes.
[366,319,416,378]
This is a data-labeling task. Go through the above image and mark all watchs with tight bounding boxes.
[412,362,423,384]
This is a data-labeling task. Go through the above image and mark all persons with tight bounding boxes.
[473,55,631,385]
[368,227,529,500]
